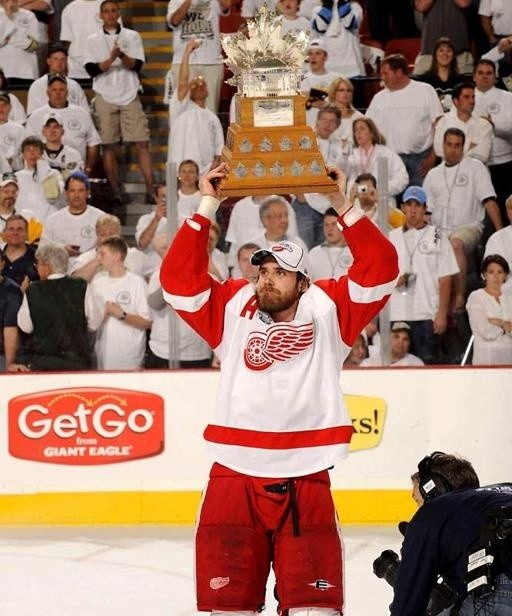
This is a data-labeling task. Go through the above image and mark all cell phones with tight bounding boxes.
[71,245,80,251]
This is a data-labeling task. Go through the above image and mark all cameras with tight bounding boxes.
[358,185,368,195]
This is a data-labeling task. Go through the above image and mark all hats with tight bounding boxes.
[0,91,10,104]
[249,239,309,278]
[402,186,427,203]
[42,111,62,126]
[49,46,67,57]
[0,171,19,189]
[391,320,412,331]
[47,72,67,85]
[308,39,327,51]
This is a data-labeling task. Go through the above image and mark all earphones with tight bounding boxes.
[481,273,485,280]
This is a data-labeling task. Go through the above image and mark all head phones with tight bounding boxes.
[418,455,451,500]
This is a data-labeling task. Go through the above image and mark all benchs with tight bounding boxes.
[82,1,175,171]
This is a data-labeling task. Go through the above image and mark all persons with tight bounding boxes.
[159,163,399,616]
[0,1,511,367]
[390,452,512,616]
